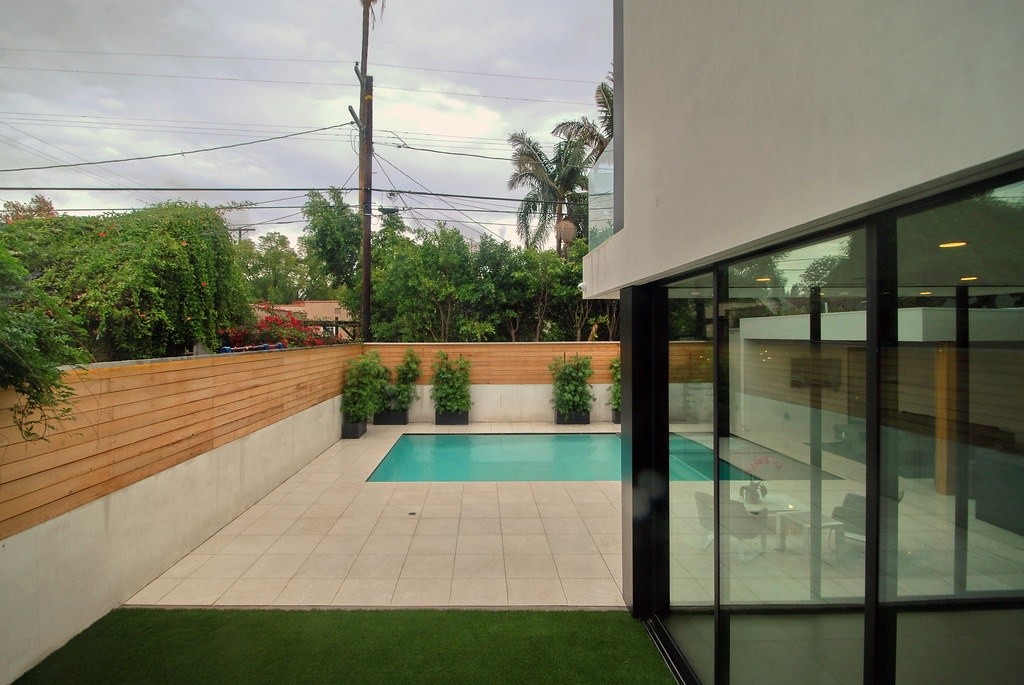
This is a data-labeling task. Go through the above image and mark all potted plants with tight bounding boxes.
[339,349,394,439]
[547,351,596,425]
[427,350,474,425]
[372,349,423,424]
[605,357,621,424]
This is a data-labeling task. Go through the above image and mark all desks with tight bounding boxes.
[778,510,845,562]
[737,496,794,535]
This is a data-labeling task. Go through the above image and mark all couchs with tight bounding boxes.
[836,410,1024,535]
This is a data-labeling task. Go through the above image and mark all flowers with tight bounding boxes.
[739,455,784,499]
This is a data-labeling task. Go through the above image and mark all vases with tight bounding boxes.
[745,490,761,504]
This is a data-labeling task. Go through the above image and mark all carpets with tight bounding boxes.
[802,439,935,478]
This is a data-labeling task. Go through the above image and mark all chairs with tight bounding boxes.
[828,490,905,556]
[695,491,768,563]
